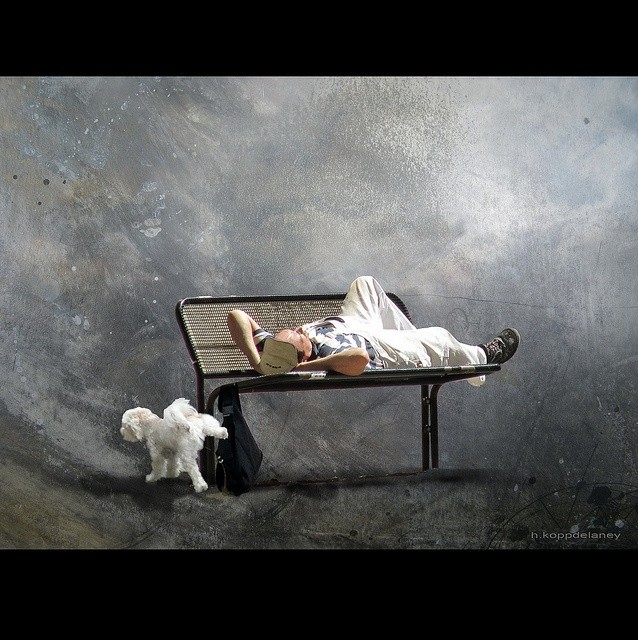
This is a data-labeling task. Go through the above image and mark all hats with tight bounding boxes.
[258,337,298,376]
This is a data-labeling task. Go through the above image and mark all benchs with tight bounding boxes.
[175,292,501,487]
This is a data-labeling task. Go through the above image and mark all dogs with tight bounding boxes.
[120,397,230,493]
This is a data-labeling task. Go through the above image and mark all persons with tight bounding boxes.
[227,275,520,388]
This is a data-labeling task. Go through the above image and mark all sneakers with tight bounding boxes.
[477,328,519,365]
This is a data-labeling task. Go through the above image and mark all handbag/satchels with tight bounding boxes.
[215,382,263,498]
